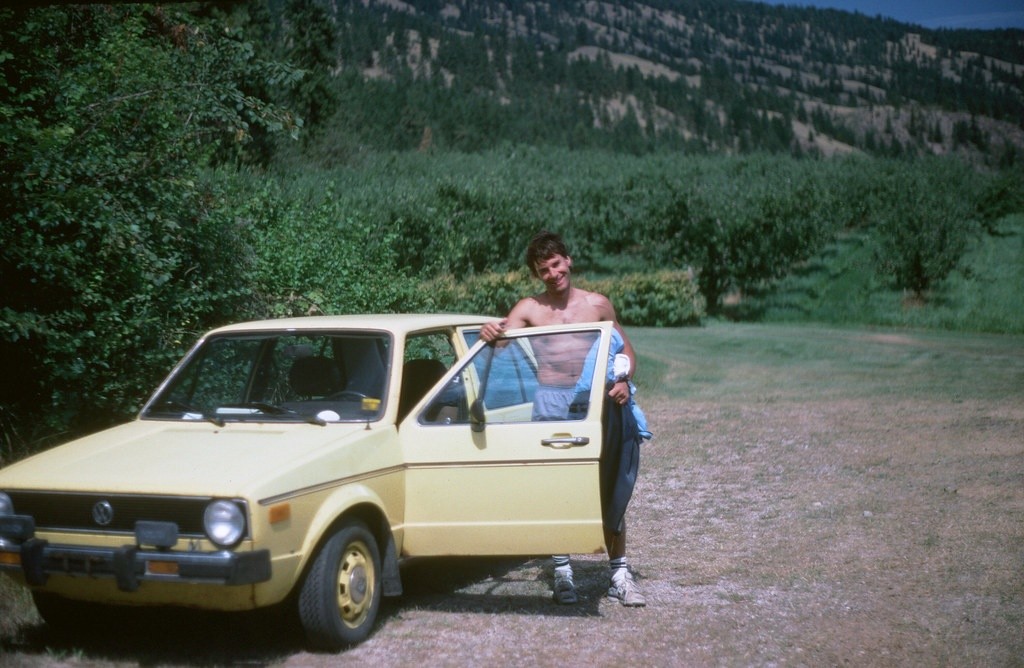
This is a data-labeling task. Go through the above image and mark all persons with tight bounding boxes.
[479,233,645,608]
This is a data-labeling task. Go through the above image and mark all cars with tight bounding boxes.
[0,313,613,649]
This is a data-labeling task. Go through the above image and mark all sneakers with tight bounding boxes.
[555,569,577,604]
[608,572,645,607]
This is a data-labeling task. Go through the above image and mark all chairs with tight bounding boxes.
[394,361,456,432]
[282,357,349,412]
